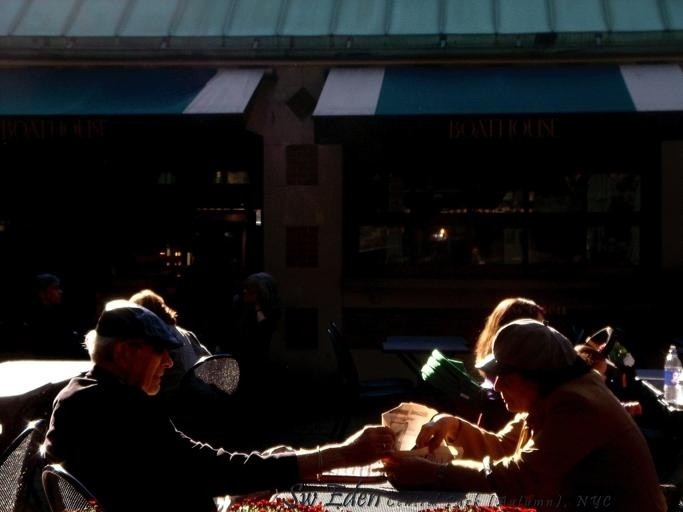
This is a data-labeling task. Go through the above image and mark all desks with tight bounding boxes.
[0,355,98,406]
[267,441,501,510]
[630,365,683,423]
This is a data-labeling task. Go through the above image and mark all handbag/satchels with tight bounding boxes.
[195,355,241,394]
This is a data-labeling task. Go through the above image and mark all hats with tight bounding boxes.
[474,317,577,375]
[96,300,184,350]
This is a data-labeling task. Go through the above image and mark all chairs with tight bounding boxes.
[36,464,98,512]
[1,416,44,510]
[184,356,243,399]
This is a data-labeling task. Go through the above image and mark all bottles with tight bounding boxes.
[664,346,682,405]
